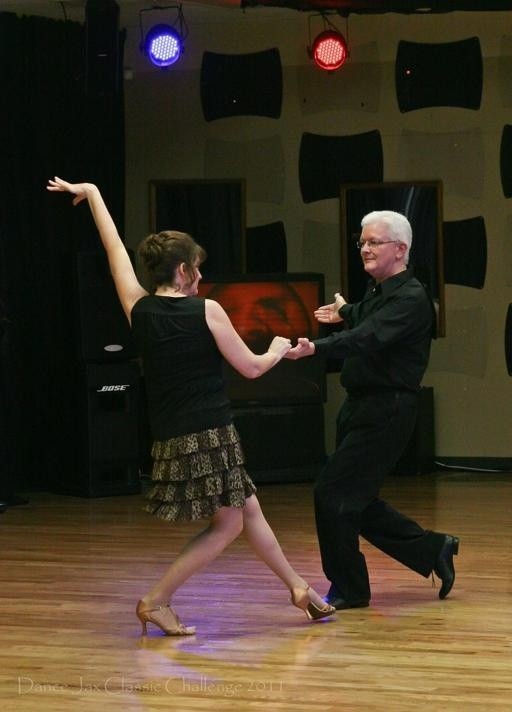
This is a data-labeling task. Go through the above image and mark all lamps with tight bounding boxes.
[300,5,352,73]
[140,0,187,69]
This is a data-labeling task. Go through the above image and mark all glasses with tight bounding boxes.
[357,239,399,251]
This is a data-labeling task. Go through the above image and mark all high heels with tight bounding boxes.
[136,599,196,635]
[290,584,336,620]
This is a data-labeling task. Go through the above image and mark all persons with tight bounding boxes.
[46,174,336,636]
[283,207,461,610]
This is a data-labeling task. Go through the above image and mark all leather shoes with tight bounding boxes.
[322,595,371,609]
[433,534,459,598]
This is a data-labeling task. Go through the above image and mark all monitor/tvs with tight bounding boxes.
[193,271,326,406]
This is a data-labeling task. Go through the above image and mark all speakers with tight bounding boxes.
[59,247,137,363]
[43,364,143,498]
[386,385,435,477]
